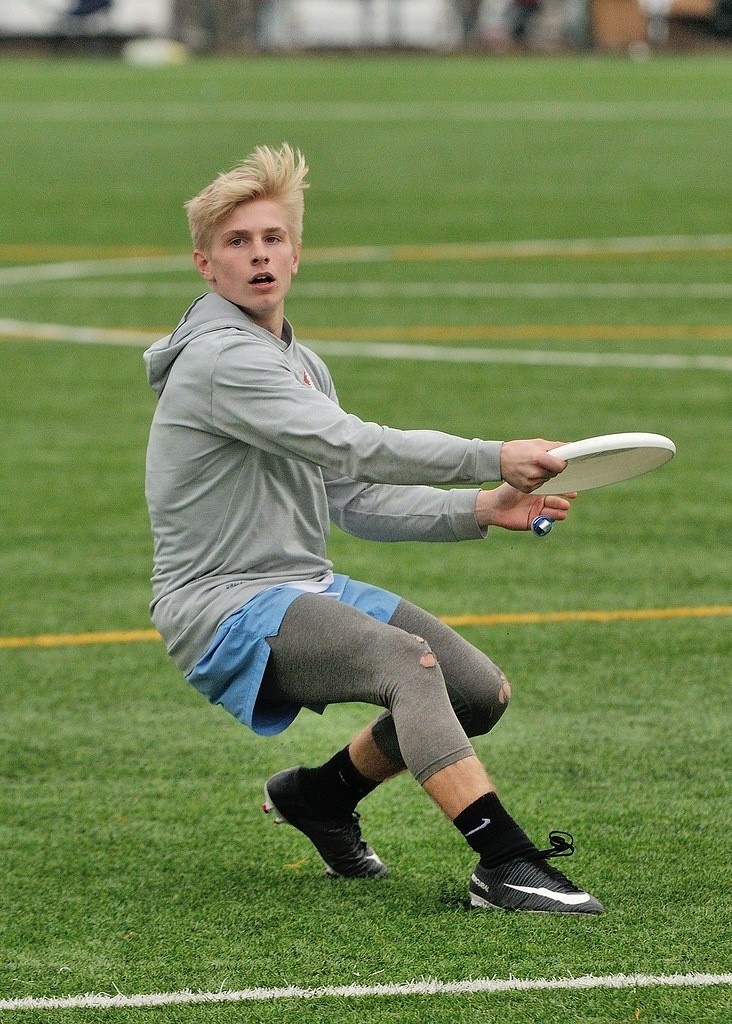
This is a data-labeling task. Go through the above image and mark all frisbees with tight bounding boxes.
[526,432,676,495]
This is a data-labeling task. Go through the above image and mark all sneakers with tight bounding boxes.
[263,766,388,879]
[469,831,604,916]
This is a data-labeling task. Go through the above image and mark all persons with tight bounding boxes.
[144,141,608,914]
